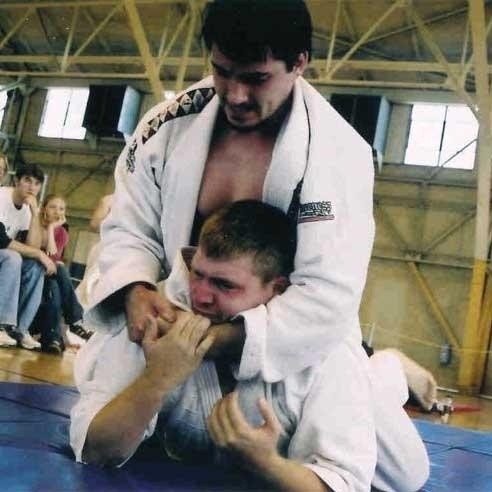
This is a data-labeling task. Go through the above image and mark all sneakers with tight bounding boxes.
[45,340,62,353]
[70,323,93,339]
[0,328,17,348]
[9,326,41,352]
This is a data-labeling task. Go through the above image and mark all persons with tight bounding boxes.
[0,153,10,181]
[72,2,430,492]
[1,163,59,352]
[70,197,438,492]
[38,194,94,353]
